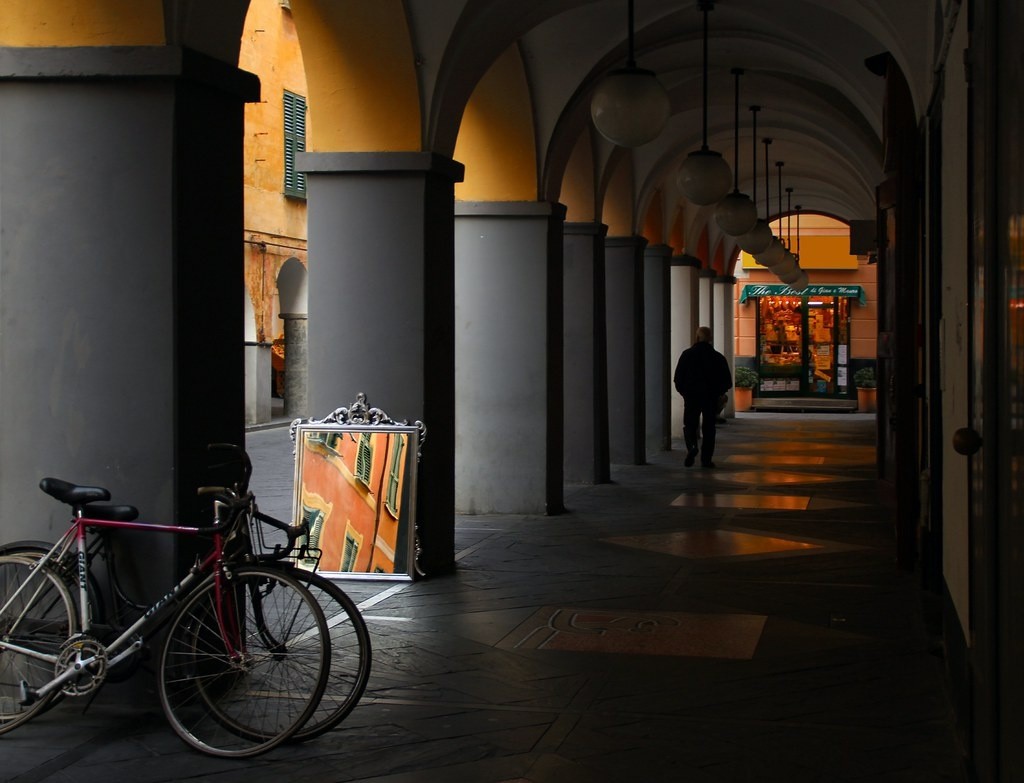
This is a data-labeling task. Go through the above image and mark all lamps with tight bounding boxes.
[737,106,772,254]
[753,138,786,265]
[676,1,732,205]
[788,270,808,290]
[769,188,795,276]
[715,67,757,236]
[593,0,670,148]
[779,205,802,283]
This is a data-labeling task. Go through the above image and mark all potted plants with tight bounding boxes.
[734,365,761,412]
[852,366,878,413]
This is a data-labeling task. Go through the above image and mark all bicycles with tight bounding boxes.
[1,444,372,760]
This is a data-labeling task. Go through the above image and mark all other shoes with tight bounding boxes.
[701,460,715,468]
[685,445,699,467]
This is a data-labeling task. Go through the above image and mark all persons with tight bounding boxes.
[673,327,732,467]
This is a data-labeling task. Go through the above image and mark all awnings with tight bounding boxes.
[742,284,860,298]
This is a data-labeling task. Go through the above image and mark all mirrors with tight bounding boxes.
[289,394,427,584]
